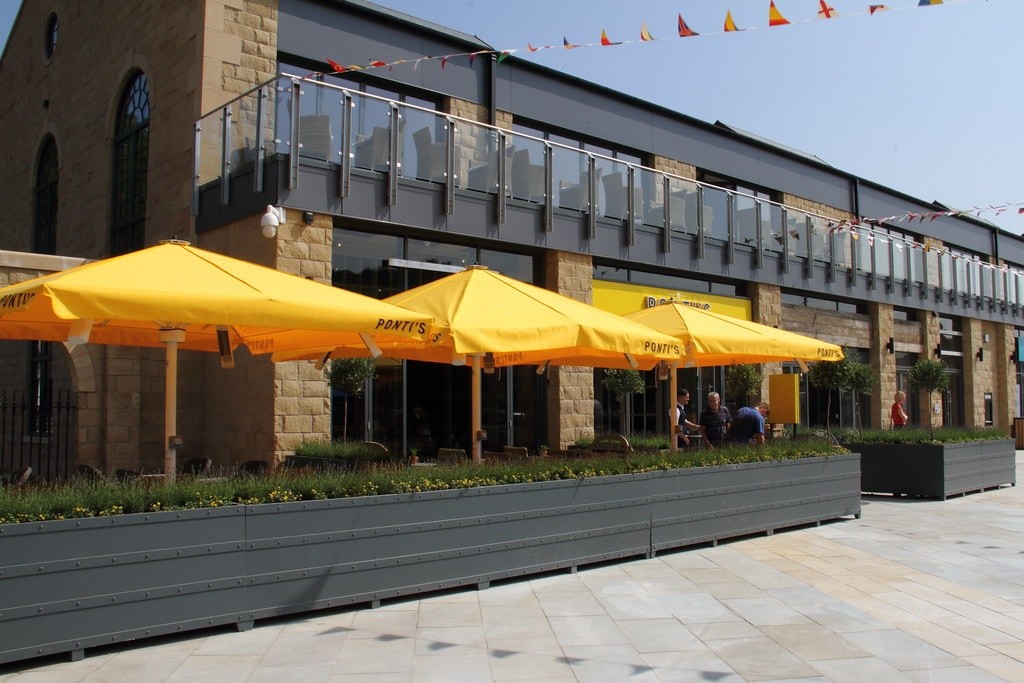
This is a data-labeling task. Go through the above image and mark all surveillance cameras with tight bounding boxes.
[261,213,279,238]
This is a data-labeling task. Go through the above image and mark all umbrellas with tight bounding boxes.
[514,298,845,452]
[0,237,434,491]
[271,261,686,464]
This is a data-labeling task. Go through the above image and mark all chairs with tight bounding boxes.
[9,457,271,488]
[354,440,529,467]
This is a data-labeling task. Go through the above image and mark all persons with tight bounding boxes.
[667,387,700,453]
[701,391,733,453]
[892,391,908,430]
[726,402,769,447]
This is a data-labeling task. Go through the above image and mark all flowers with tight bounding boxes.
[0,435,852,526]
[788,424,1010,442]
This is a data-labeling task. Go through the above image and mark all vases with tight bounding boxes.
[838,439,1017,500]
[0,451,864,666]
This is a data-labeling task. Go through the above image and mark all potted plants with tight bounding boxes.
[537,444,550,457]
[285,435,364,472]
[408,447,419,465]
